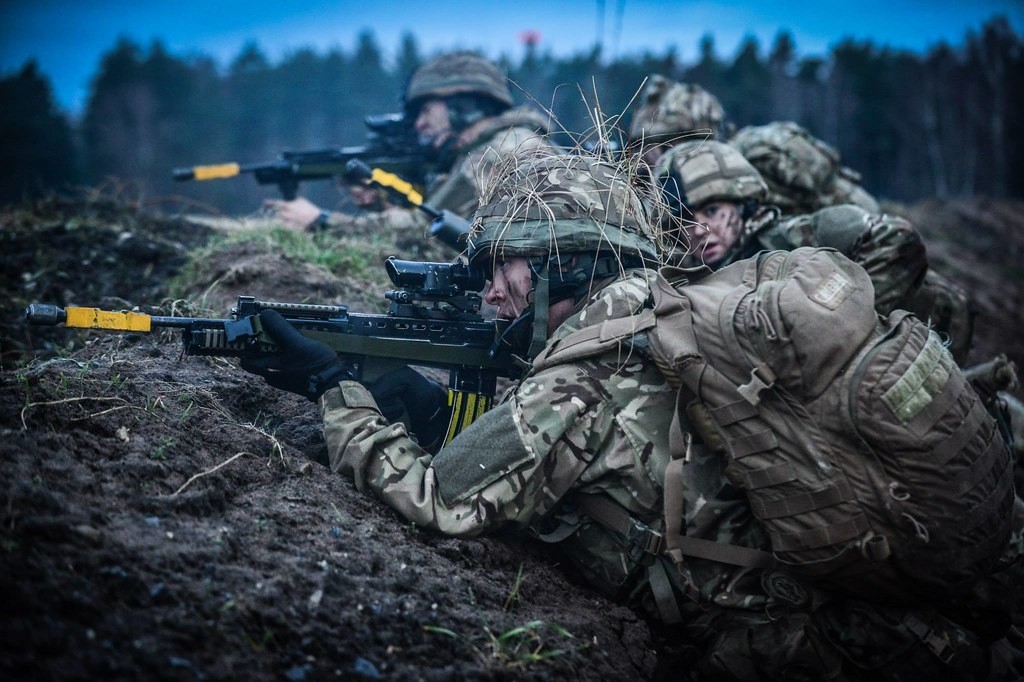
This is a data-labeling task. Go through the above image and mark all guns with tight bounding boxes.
[171,113,438,209]
[23,256,522,451]
[347,158,472,255]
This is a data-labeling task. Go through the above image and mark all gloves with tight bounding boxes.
[238,309,351,404]
[359,357,451,445]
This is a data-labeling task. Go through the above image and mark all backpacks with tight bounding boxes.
[525,247,1016,580]
[737,119,883,223]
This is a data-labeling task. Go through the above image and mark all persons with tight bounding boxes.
[240,50,1024,680]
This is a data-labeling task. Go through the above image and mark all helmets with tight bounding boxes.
[629,75,726,142]
[650,140,769,225]
[404,51,514,108]
[468,154,658,261]
